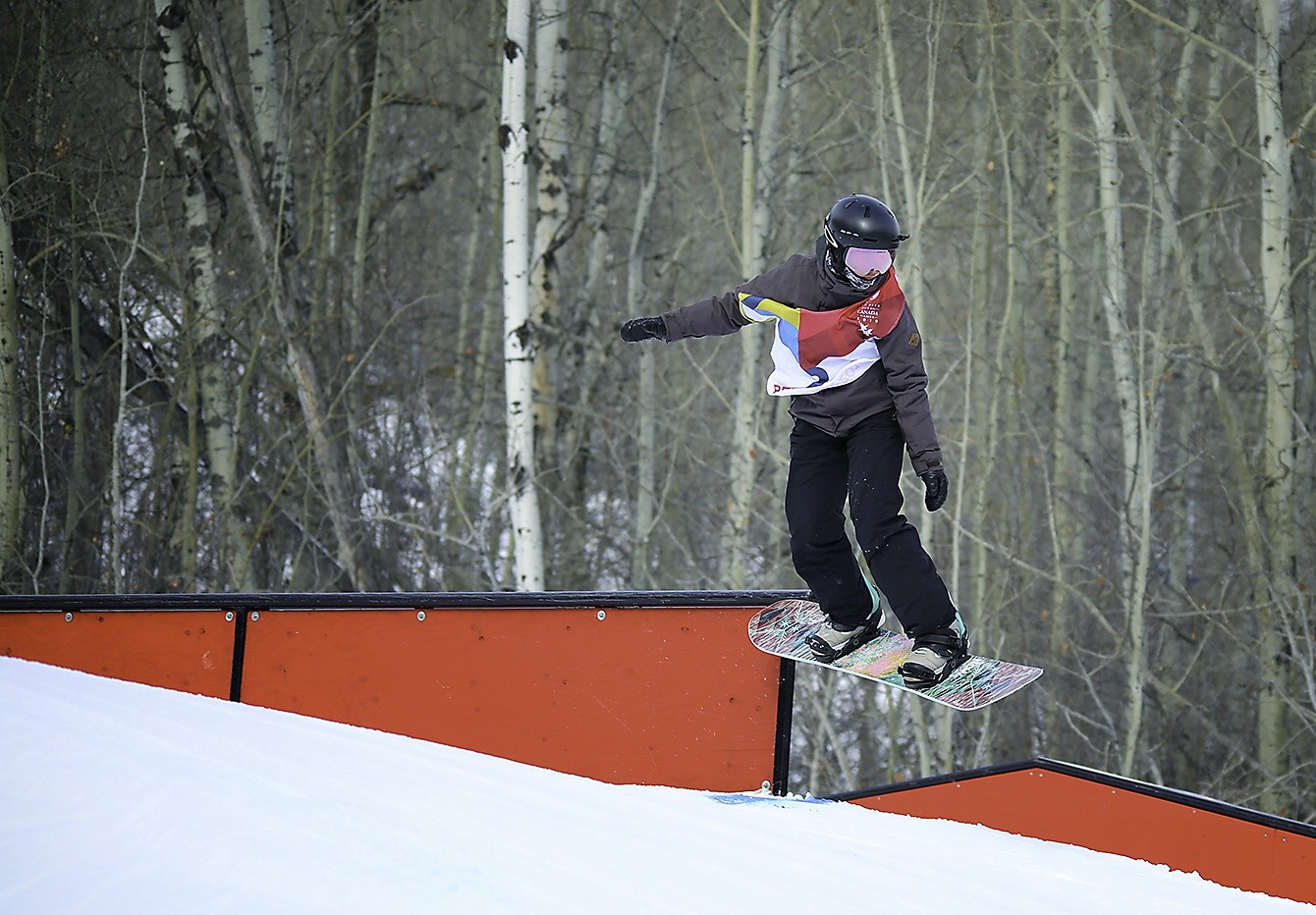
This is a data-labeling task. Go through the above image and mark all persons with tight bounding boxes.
[619,194,970,690]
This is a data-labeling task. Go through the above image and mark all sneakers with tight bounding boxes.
[810,607,886,657]
[904,620,970,674]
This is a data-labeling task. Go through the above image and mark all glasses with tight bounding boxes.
[842,246,897,276]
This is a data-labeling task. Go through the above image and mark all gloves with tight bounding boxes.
[921,470,948,512]
[621,318,665,344]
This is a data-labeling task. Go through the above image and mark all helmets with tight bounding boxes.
[824,194,900,292]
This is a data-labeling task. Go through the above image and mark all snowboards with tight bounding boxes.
[746,598,1047,712]
